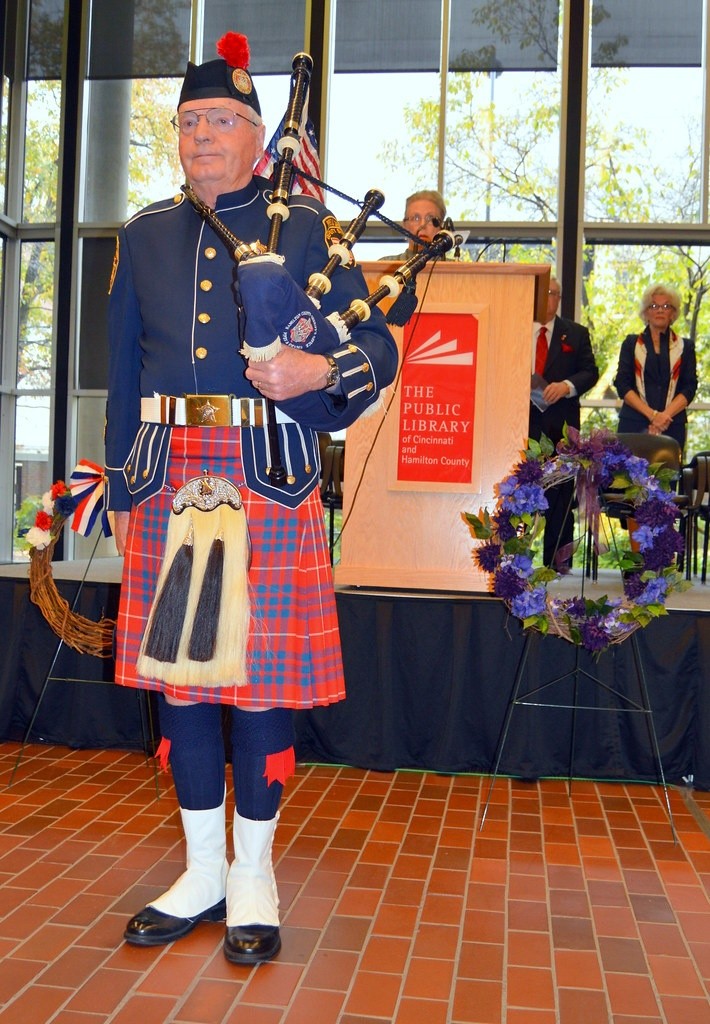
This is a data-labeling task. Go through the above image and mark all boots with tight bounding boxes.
[226,808,281,964]
[124,783,230,945]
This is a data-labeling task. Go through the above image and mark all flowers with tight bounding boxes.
[216,32,250,70]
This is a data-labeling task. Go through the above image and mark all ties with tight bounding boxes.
[535,328,548,378]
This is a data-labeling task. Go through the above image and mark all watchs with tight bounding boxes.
[323,353,339,386]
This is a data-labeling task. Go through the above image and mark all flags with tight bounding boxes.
[255,109,324,204]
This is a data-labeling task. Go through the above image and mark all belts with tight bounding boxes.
[140,392,297,428]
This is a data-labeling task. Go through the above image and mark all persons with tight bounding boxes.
[103,58,399,963]
[378,190,446,260]
[528,276,600,575]
[614,283,698,551]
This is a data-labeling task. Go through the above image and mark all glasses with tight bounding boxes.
[646,303,676,311]
[548,289,558,296]
[171,107,258,135]
[404,214,441,223]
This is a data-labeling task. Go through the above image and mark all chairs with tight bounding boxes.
[577,433,710,588]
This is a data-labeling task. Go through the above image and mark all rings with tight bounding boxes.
[257,382,259,388]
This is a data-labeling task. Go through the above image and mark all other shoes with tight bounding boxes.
[554,561,573,577]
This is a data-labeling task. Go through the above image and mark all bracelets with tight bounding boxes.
[650,410,658,423]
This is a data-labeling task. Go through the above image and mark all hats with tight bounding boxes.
[178,31,262,117]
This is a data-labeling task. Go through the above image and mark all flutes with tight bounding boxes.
[179,50,455,487]
